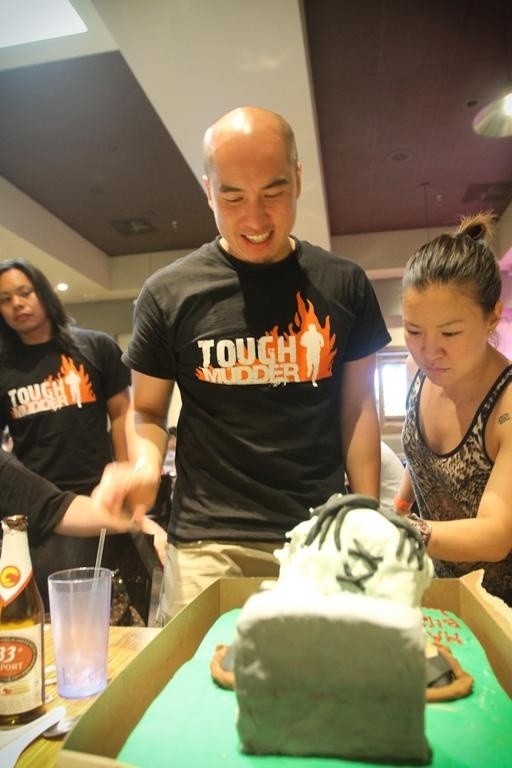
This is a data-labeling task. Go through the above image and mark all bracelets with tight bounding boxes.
[395,498,409,511]
[414,516,431,546]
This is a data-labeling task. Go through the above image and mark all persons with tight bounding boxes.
[89,104,393,626]
[0,452,169,571]
[160,428,177,497]
[378,442,405,510]
[394,210,512,610]
[1,256,157,626]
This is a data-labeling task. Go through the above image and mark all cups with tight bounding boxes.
[45,566,112,699]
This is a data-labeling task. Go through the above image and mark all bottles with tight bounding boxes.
[2,514,49,728]
